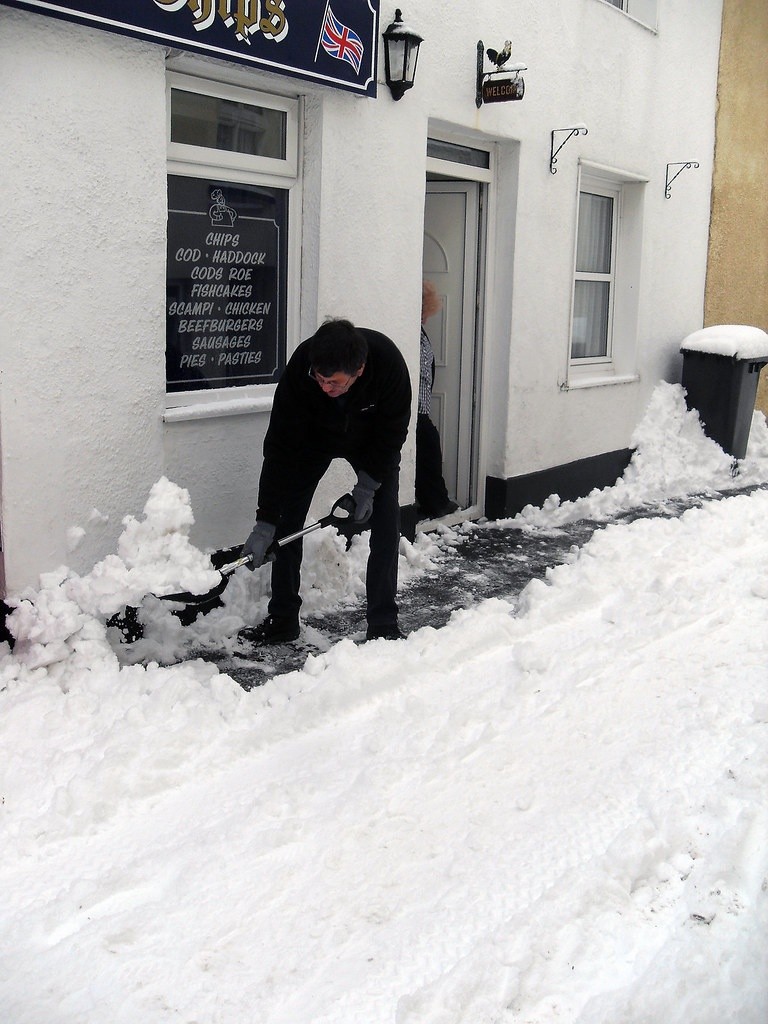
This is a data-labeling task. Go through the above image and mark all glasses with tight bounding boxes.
[308,363,356,391]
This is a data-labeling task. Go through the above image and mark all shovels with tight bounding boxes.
[152,493,373,602]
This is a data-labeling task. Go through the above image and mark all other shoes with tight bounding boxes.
[434,499,459,517]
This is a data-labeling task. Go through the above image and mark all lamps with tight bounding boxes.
[376,8,422,100]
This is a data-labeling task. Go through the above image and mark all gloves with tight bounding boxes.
[240,520,276,572]
[351,472,381,524]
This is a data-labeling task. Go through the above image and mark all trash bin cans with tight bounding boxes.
[678,346,768,478]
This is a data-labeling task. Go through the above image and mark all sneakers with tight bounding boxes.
[367,622,407,641]
[237,613,300,646]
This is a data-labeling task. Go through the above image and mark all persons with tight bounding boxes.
[238,315,412,648]
[415,281,458,518]
